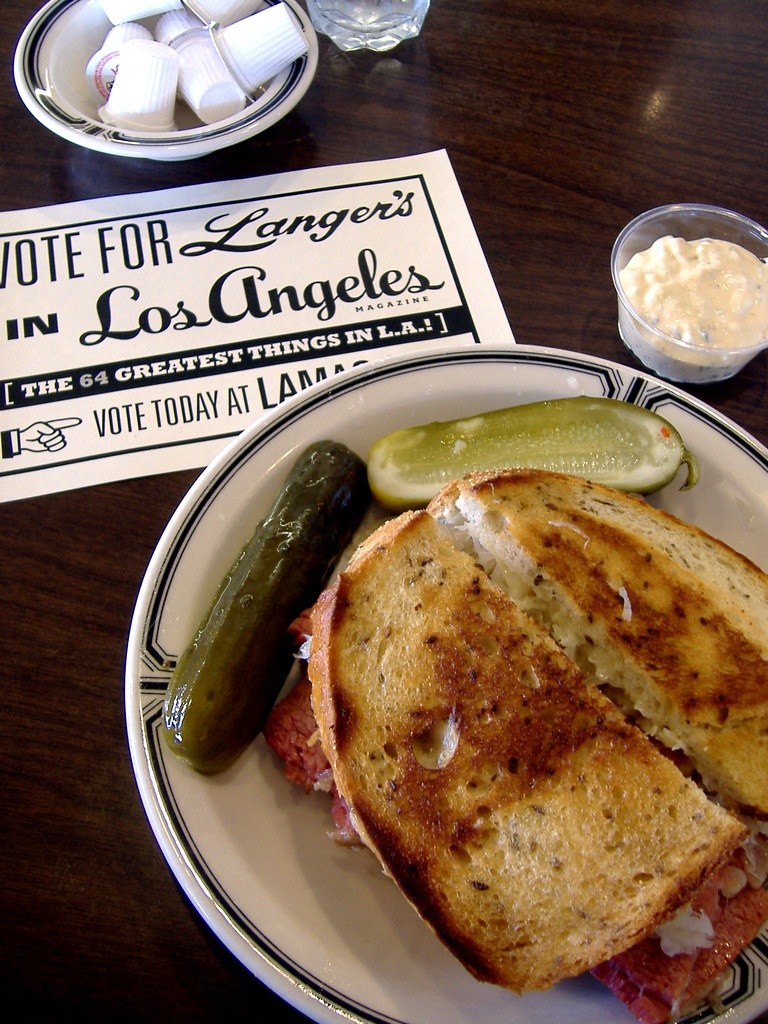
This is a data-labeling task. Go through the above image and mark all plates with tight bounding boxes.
[122,341,768,1023]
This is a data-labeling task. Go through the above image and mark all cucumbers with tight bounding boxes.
[160,439,371,776]
[367,397,699,511]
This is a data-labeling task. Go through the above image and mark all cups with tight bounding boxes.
[304,1,431,53]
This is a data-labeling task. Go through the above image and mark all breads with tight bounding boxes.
[426,468,767,831]
[307,510,753,992]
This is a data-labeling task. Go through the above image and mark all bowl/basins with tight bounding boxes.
[12,2,319,161]
[608,201,768,383]
